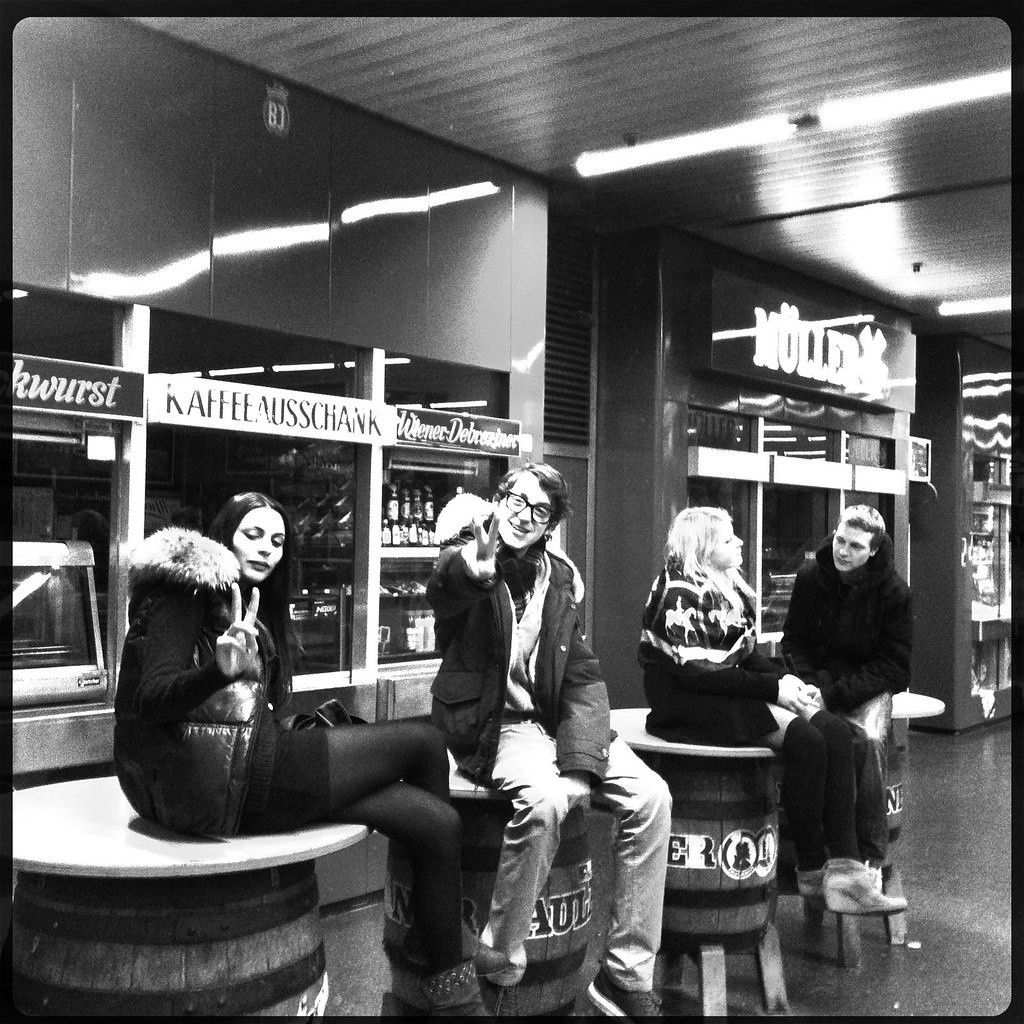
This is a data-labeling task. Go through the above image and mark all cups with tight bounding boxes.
[378,611,435,655]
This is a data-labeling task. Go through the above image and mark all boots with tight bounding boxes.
[397,908,510,976]
[381,959,492,1016]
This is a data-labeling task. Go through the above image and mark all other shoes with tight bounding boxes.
[793,858,909,915]
[584,964,665,1016]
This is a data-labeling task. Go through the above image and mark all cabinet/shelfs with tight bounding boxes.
[379,547,442,662]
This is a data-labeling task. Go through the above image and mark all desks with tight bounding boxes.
[610,708,789,1017]
[13,777,367,1017]
[383,747,591,1016]
[776,691,945,969]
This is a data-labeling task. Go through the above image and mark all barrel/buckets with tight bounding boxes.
[380,797,594,1017]
[611,749,782,953]
[11,871,330,1024]
[777,715,909,894]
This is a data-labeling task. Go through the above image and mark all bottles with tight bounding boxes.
[378,487,471,549]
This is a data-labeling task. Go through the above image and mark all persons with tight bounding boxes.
[425,461,672,1017]
[637,506,908,917]
[113,491,488,1016]
[780,503,915,774]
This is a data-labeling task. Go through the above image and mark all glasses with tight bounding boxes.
[506,491,554,524]
